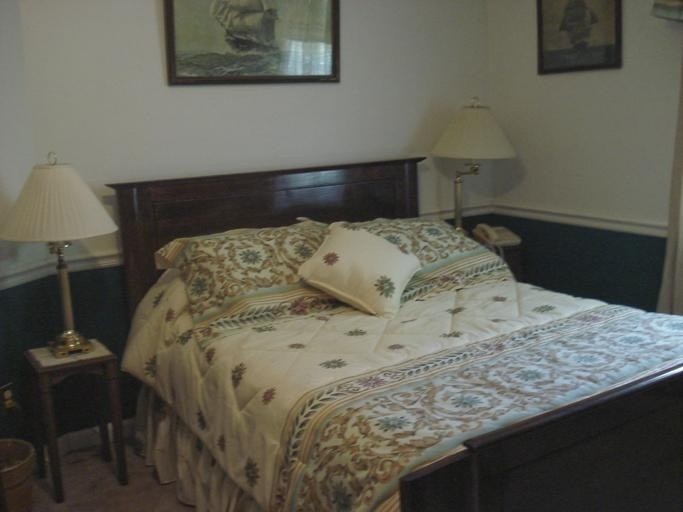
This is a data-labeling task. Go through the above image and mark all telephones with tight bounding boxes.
[472,224,522,247]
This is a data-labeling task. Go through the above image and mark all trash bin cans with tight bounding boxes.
[0,439,35,512]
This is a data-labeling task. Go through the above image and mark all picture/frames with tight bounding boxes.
[161,0,341,88]
[534,0,623,76]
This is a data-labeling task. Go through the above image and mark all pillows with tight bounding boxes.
[154,220,343,324]
[344,215,513,302]
[299,224,420,319]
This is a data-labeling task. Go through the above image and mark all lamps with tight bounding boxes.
[430,96,520,236]
[0,151,120,360]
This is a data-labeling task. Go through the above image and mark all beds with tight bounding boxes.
[101,157,683,512]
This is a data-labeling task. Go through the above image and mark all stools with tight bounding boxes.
[22,338,127,504]
